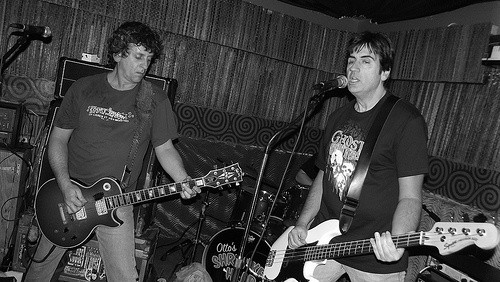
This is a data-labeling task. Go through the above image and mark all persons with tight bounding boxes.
[295,151,320,187]
[288,31,428,282]
[23,21,201,282]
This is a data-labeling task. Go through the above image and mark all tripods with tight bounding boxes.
[162,188,209,265]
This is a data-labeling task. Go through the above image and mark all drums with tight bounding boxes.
[228,183,294,229]
[283,185,310,226]
[202,216,292,282]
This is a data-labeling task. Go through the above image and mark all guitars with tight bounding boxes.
[33,159,246,249]
[262,211,500,282]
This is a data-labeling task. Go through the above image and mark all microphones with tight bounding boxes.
[312,75,349,91]
[10,23,51,38]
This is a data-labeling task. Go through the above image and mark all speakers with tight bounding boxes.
[31,98,157,237]
[0,143,33,272]
